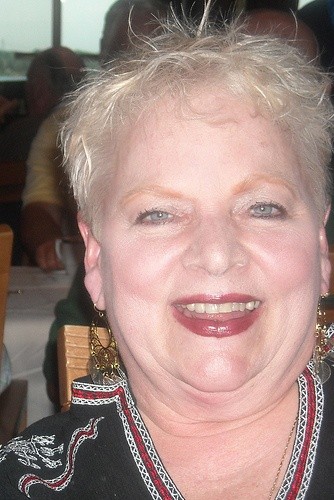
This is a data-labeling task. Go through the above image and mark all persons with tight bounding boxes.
[0,0,334,270]
[0,0,334,500]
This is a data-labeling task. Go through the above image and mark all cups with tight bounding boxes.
[54,235,87,277]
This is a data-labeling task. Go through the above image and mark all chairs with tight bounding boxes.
[57,323,110,411]
[0,224,28,446]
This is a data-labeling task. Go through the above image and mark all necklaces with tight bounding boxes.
[267,412,298,500]
